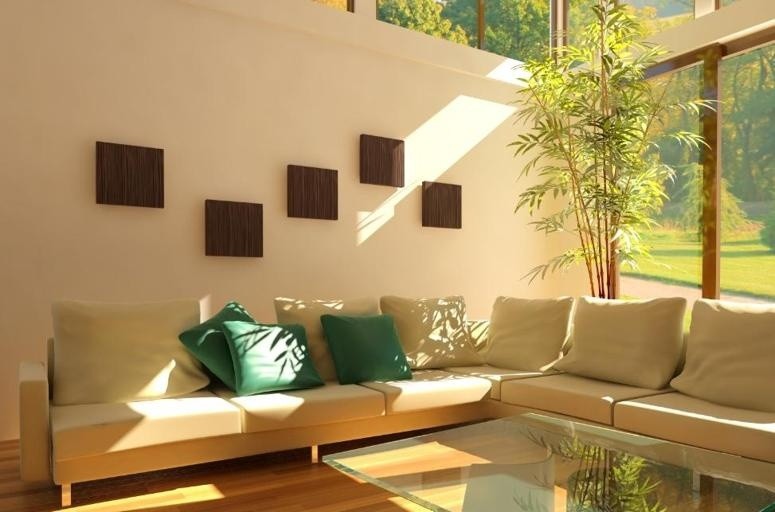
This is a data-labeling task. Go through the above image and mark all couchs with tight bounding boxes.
[441,317,775,462]
[19,336,490,508]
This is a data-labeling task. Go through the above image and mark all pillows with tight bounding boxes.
[272,298,379,382]
[542,294,688,390]
[320,313,413,386]
[669,296,775,414]
[378,294,484,371]
[47,298,210,406]
[222,319,325,396]
[179,302,259,392]
[478,294,574,372]
